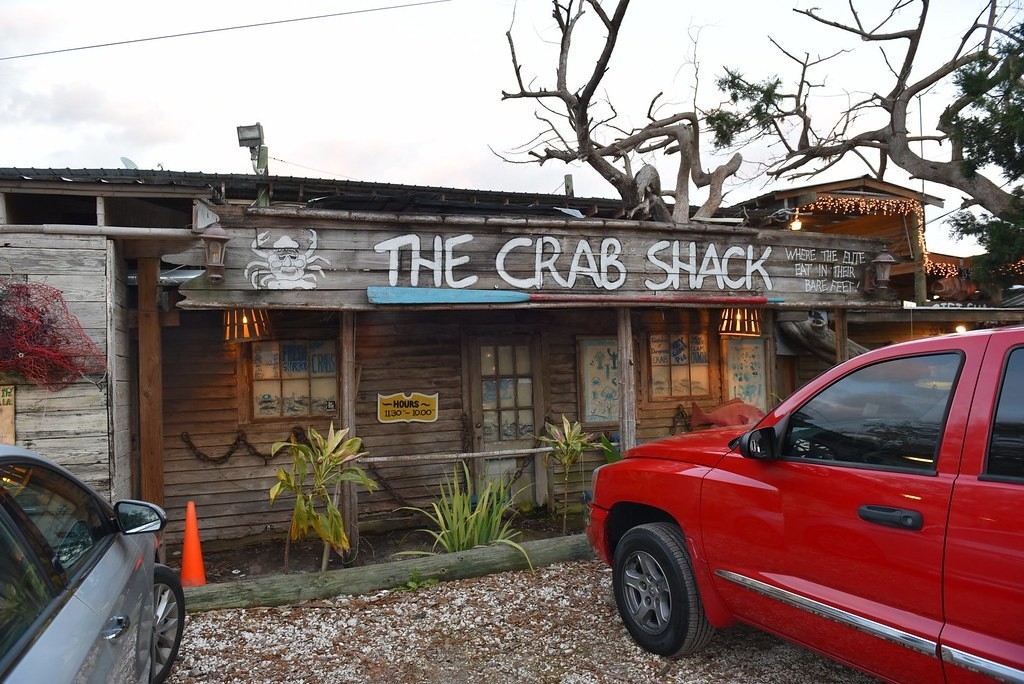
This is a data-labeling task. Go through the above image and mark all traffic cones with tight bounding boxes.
[178,497,209,589]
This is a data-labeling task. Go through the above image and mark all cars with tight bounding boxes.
[0,445,191,684]
[584,321,1024,684]
